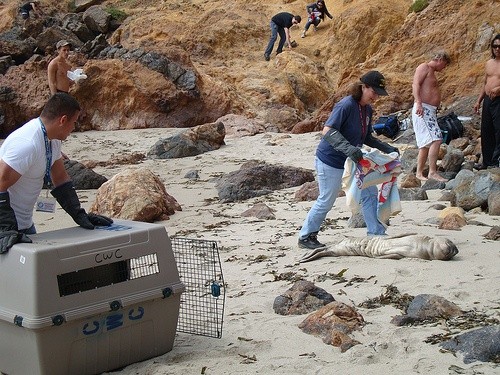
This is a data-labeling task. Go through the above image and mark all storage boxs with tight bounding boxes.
[0,218,186,375]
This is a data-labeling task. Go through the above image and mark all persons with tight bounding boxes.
[302,0,334,38]
[0,92,114,254]
[412,50,452,182]
[48,39,76,98]
[264,12,302,61]
[298,71,401,249]
[474,34,500,171]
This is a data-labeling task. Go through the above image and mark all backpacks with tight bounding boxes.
[373,114,401,140]
[436,112,464,144]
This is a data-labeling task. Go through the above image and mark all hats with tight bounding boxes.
[56,40,72,49]
[361,71,388,95]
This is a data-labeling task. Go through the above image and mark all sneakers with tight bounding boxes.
[298,231,325,249]
[367,232,387,236]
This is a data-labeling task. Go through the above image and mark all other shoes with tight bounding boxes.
[302,32,305,38]
[473,165,487,172]
[313,27,318,32]
[264,52,270,60]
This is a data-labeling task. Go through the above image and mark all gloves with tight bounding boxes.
[50,181,114,230]
[0,191,32,254]
[365,134,400,158]
[324,128,363,163]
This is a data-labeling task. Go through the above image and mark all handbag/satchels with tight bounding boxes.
[397,113,413,130]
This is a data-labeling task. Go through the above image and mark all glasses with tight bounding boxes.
[294,22,297,24]
[367,88,377,95]
[318,4,323,6]
[493,45,500,48]
